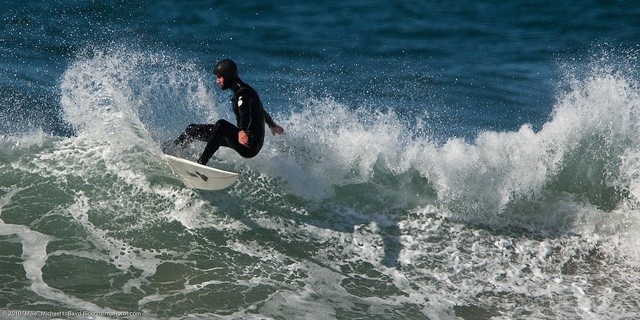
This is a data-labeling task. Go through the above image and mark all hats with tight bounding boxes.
[213,58,238,91]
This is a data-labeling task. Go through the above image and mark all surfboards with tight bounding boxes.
[161,151,239,192]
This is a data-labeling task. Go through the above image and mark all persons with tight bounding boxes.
[161,59,286,165]
